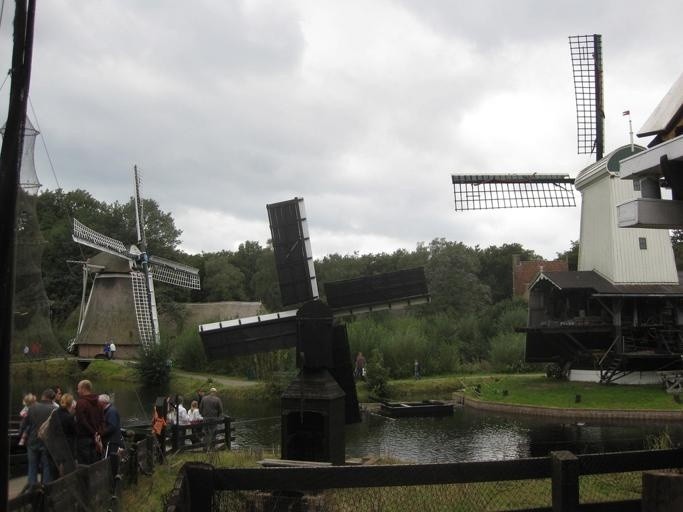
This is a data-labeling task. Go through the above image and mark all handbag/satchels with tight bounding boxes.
[36,416,52,448]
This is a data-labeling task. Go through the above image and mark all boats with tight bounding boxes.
[378,402,454,417]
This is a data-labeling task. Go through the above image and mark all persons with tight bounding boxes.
[103,341,109,361]
[21,343,31,362]
[30,340,39,362]
[169,387,223,453]
[16,379,121,486]
[413,358,421,380]
[354,350,367,380]
[108,340,116,360]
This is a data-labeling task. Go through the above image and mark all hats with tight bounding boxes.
[207,387,218,395]
